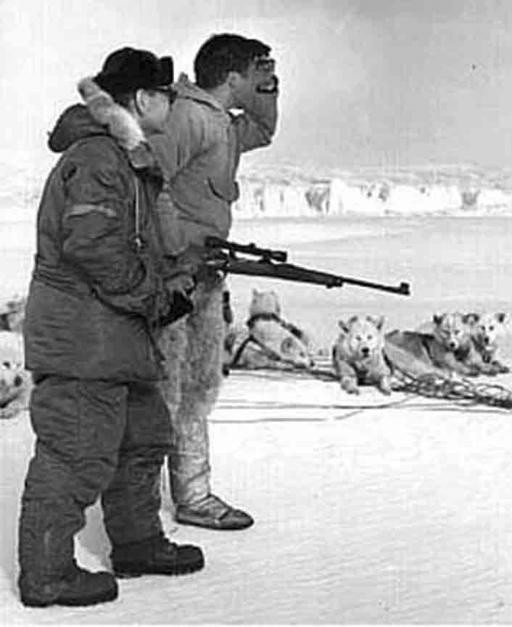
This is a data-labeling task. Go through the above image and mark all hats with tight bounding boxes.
[92,47,174,96]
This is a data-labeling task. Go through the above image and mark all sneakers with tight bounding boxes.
[15,568,118,608]
[110,535,204,579]
[176,494,254,531]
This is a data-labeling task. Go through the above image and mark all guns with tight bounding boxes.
[163,236,410,297]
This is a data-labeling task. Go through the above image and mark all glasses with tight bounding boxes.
[143,83,177,104]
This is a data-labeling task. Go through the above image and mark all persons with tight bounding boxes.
[16,45,207,609]
[141,32,282,535]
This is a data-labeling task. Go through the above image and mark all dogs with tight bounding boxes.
[223,280,316,374]
[0,299,34,419]
[331,312,509,395]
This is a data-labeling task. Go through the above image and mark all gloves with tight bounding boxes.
[152,290,195,329]
